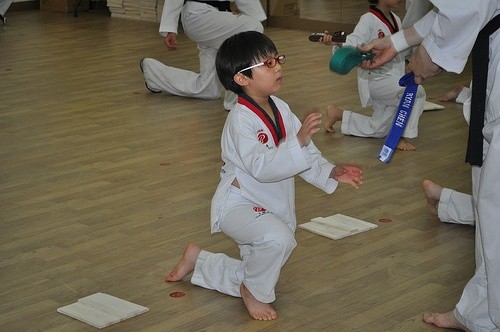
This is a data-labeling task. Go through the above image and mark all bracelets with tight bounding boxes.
[391,29,409,53]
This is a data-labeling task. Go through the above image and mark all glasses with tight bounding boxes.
[238,55,286,74]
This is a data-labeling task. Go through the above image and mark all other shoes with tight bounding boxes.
[140,59,161,93]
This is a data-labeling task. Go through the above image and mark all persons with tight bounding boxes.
[140,0,267,111]
[164,29,362,320]
[319,0,500,332]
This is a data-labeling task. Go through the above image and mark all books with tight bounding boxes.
[57,292,149,329]
